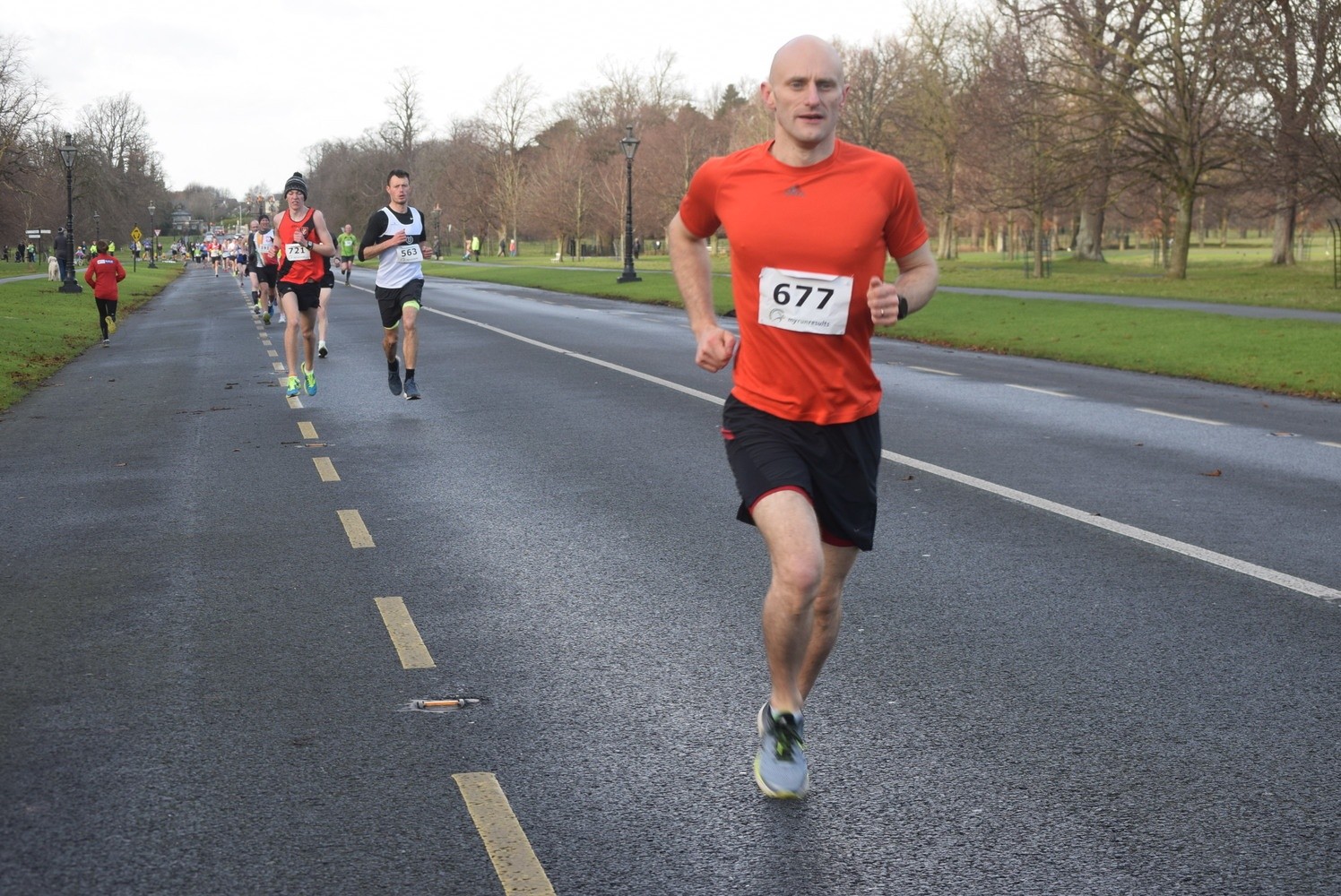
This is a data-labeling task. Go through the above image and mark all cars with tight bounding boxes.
[203,225,226,245]
[233,233,241,239]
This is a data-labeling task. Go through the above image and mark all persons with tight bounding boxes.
[357,169,434,400]
[84,240,126,348]
[267,171,337,398]
[631,237,661,260]
[171,215,342,358]
[666,35,941,800]
[431,234,516,263]
[337,225,359,287]
[0,236,163,263]
[54,227,67,282]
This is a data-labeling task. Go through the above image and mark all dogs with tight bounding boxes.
[47,256,61,281]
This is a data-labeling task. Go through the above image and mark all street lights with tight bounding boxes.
[147,201,158,268]
[614,124,643,284]
[428,204,444,261]
[57,132,82,293]
[93,210,100,242]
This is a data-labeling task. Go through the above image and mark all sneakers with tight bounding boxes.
[754,700,809,798]
[285,376,301,398]
[388,356,402,396]
[301,362,317,397]
[403,378,421,399]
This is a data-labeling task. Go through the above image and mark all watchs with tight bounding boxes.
[306,240,313,250]
[896,292,908,320]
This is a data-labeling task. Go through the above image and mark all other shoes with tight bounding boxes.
[257,298,263,308]
[254,304,260,314]
[103,339,109,347]
[105,316,116,334]
[345,281,350,287]
[317,344,328,359]
[268,303,274,316]
[262,311,271,326]
[279,314,286,323]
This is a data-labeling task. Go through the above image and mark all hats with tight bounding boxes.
[57,226,65,234]
[284,172,307,201]
[258,215,270,226]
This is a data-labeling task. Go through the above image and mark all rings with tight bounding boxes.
[880,308,884,317]
